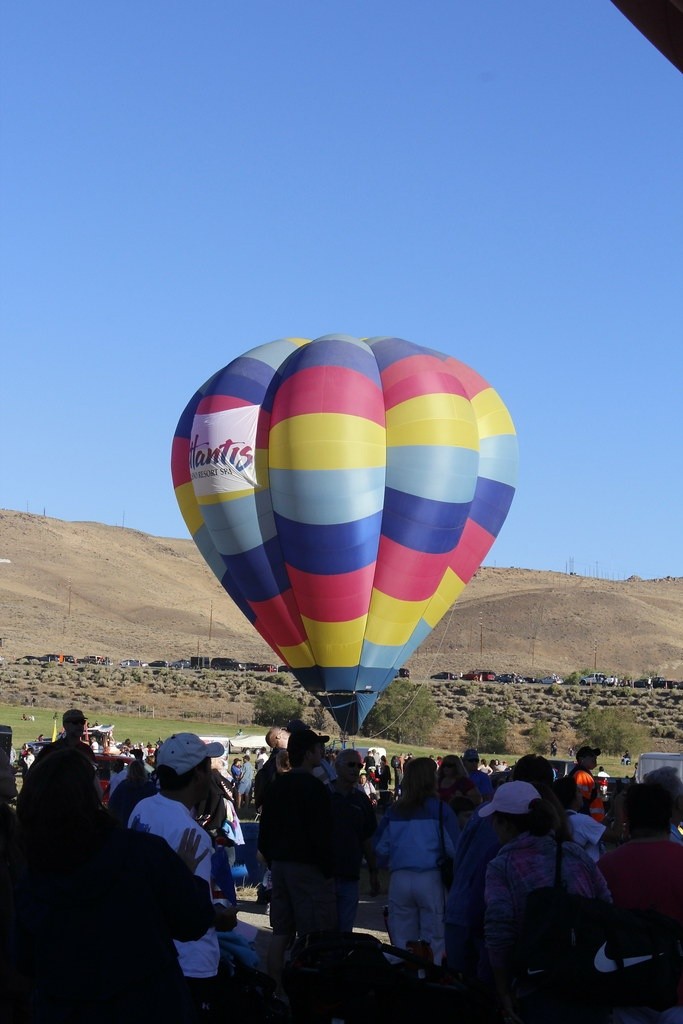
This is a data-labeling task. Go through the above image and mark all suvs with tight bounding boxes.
[211,657,247,671]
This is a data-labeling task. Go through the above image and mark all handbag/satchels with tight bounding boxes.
[510,843,683,1012]
[436,801,454,892]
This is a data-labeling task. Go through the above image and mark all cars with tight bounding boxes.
[430,672,458,681]
[398,668,410,678]
[463,669,496,681]
[495,673,565,684]
[246,662,290,672]
[149,660,169,667]
[171,659,191,668]
[16,653,114,666]
[579,673,683,689]
[118,659,143,667]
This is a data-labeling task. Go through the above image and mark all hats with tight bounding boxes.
[464,749,479,758]
[478,780,541,817]
[155,733,224,777]
[63,709,88,720]
[576,746,601,759]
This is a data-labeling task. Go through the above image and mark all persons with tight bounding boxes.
[1,708,683,1024]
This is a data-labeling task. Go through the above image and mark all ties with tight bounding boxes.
[287,730,330,752]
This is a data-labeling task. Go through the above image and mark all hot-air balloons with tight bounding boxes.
[170,332,518,742]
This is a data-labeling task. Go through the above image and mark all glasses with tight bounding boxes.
[65,720,86,726]
[590,755,597,759]
[344,762,364,769]
[442,764,454,768]
[92,763,99,772]
[466,759,479,763]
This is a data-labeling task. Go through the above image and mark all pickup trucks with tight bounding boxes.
[489,758,635,805]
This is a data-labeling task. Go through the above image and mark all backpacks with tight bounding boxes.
[281,931,411,995]
